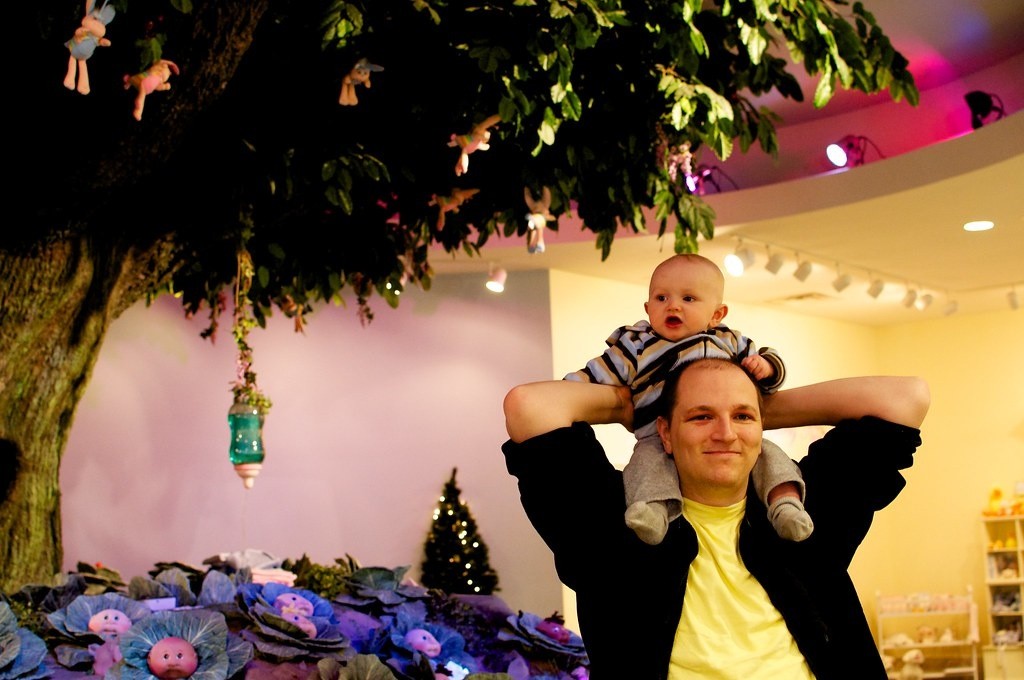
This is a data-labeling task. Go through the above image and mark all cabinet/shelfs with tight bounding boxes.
[983,514,1024,680]
[872,586,979,679]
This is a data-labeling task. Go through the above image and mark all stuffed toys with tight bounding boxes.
[447,115,501,176]
[524,186,555,254]
[339,59,384,106]
[64,0,116,95]
[123,60,180,121]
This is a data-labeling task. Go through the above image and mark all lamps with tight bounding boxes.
[965,90,993,128]
[764,243,784,275]
[793,253,812,282]
[926,292,943,316]
[1006,291,1020,309]
[903,288,918,308]
[826,134,866,167]
[485,261,507,293]
[725,241,755,277]
[941,297,960,317]
[832,264,851,293]
[685,163,721,194]
[914,294,932,310]
[866,279,884,298]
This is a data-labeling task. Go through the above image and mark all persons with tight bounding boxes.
[562,254,814,545]
[501,376,931,679]
[89,594,569,679]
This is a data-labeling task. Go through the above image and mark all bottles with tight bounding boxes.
[228,403,268,488]
